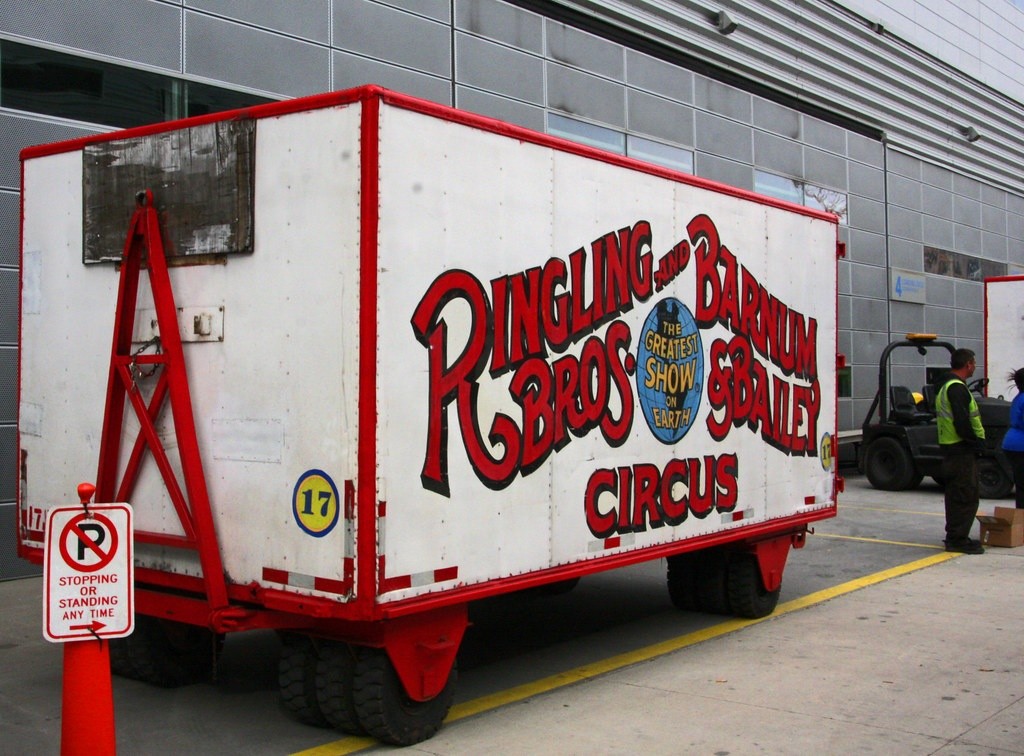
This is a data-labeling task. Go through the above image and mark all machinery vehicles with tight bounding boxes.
[858,332,1017,497]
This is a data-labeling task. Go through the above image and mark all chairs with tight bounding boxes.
[891,386,931,422]
[922,386,938,413]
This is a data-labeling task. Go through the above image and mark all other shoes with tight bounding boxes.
[946,537,984,554]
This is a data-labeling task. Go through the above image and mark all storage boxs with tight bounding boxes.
[975,506,1024,548]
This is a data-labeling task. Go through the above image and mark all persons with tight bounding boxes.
[937,348,986,554]
[1001,368,1024,509]
